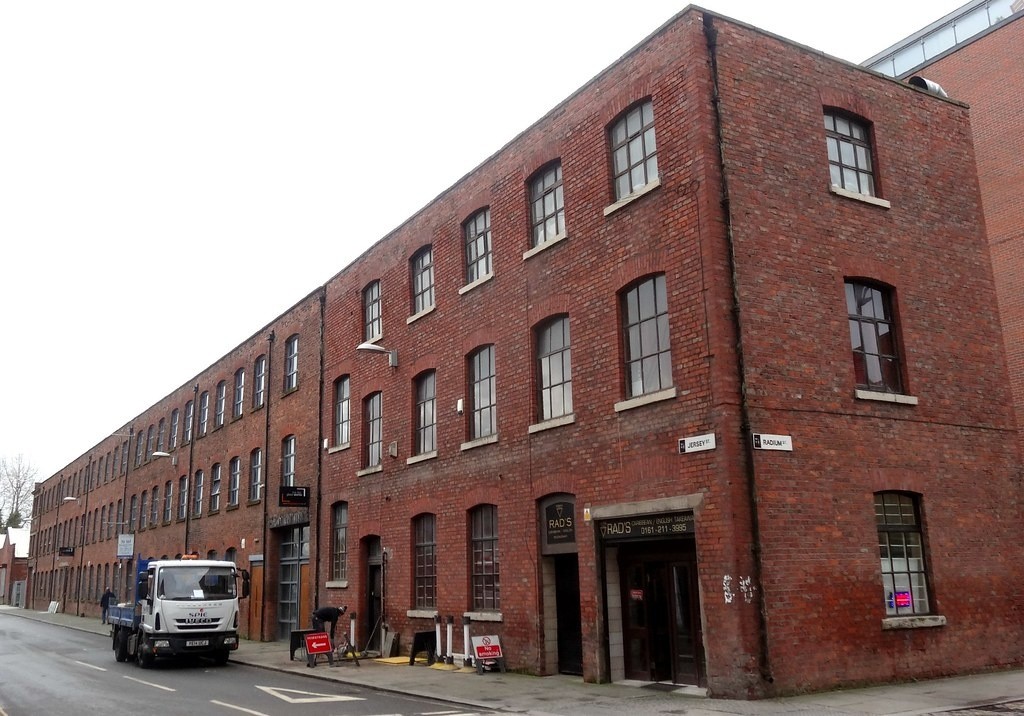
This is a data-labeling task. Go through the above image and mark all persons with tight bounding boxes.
[100,587,116,625]
[312,605,347,640]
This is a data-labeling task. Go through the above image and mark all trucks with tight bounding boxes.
[106,553,250,669]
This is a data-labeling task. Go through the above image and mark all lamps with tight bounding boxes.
[356,343,398,367]
[153,452,178,466]
[64,497,81,505]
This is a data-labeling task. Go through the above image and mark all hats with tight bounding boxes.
[339,607,345,614]
